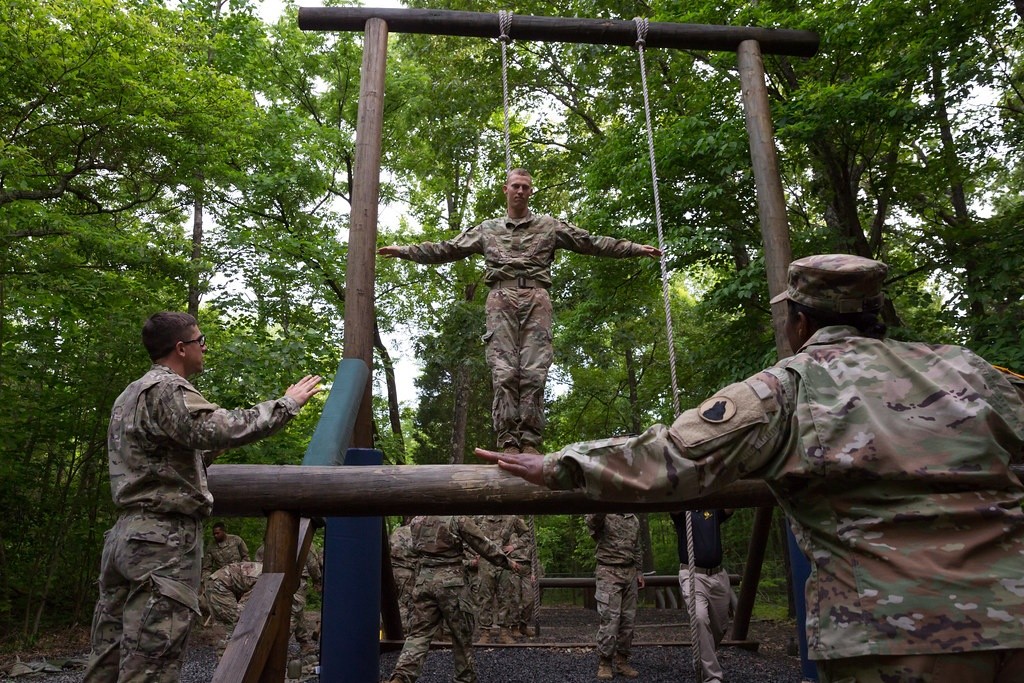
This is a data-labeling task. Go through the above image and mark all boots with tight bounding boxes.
[301,641,311,656]
[597,656,613,680]
[511,624,522,637]
[519,622,535,636]
[478,628,491,644]
[613,651,640,678]
[499,625,516,645]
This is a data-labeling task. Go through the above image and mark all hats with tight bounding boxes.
[769,253,889,313]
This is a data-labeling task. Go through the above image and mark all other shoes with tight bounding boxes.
[390,677,404,683]
[504,445,520,455]
[520,445,539,455]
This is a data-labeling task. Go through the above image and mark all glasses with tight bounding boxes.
[180,334,206,347]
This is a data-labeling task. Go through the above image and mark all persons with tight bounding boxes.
[378,167,662,454]
[387,515,535,683]
[201,523,322,655]
[474,255,1024,683]
[584,512,645,679]
[670,506,731,683]
[81,313,322,683]
